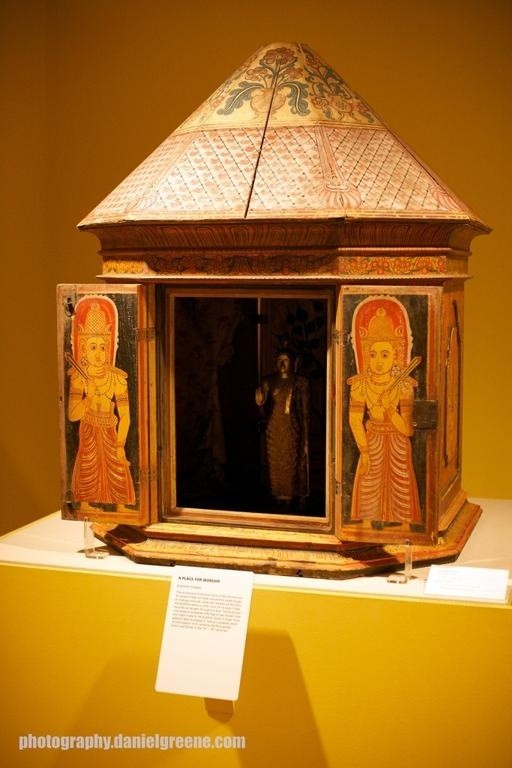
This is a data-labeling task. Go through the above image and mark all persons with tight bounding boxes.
[67,304,139,514]
[252,339,313,501]
[346,308,424,532]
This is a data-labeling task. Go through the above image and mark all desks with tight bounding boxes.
[0,508,512,768]
